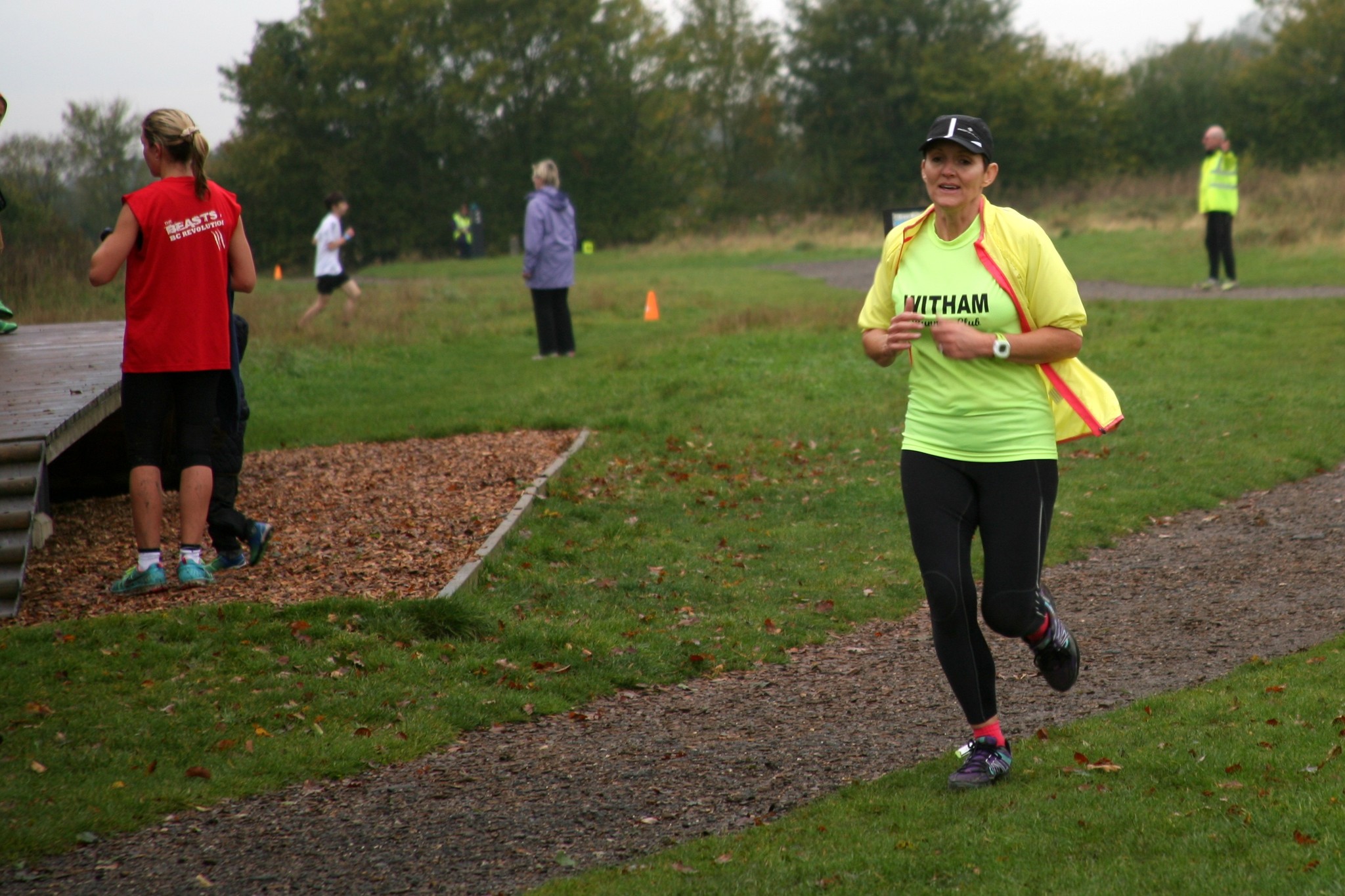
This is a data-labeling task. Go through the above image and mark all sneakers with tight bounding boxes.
[1022,580,1080,693]
[0,301,13,320]
[177,558,214,582]
[205,553,246,572]
[110,562,168,596]
[949,736,1012,787]
[0,320,17,334]
[247,522,274,566]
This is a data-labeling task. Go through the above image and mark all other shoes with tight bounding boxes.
[1200,277,1237,291]
[531,350,575,360]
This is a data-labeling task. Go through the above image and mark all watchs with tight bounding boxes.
[992,331,1013,362]
[343,233,351,243]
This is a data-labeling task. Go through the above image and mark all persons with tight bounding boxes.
[0,92,18,335]
[207,312,276,574]
[1195,121,1241,293]
[857,115,1125,789]
[451,202,475,259]
[522,156,580,363]
[294,190,364,335]
[89,108,257,596]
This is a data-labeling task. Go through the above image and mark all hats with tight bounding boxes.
[918,114,993,162]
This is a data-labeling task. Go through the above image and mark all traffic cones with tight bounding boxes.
[644,289,659,321]
[274,263,283,280]
